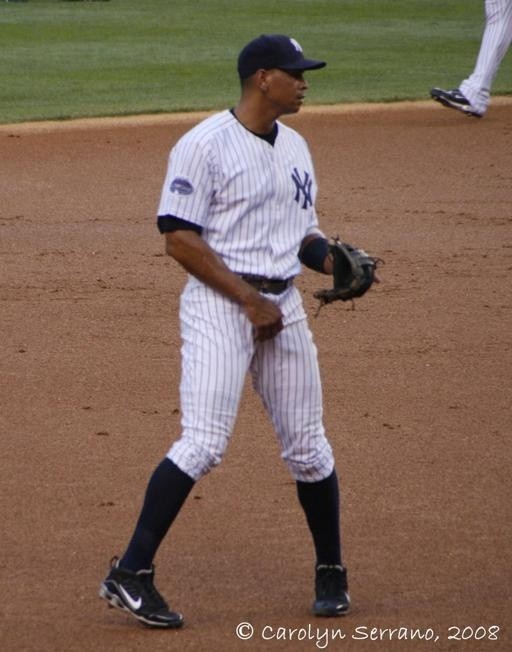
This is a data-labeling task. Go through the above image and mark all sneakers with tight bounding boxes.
[429,86,484,120]
[311,564,351,617]
[98,555,184,630]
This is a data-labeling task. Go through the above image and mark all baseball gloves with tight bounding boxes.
[314,237,384,303]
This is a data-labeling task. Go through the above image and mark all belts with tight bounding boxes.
[237,271,296,296]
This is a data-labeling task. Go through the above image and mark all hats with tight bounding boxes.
[237,32,329,80]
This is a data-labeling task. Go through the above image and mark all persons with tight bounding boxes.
[98,35,387,629]
[427,0,512,117]
